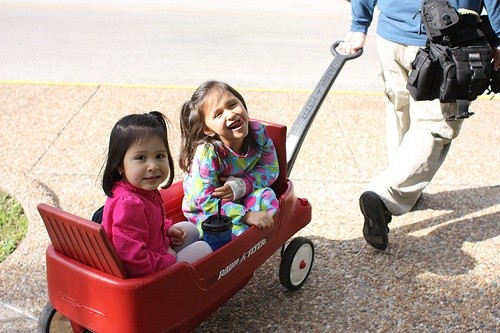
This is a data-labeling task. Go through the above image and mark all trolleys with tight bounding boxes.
[36,40,363,333]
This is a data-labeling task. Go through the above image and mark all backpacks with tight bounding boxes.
[408,0,500,119]
[359,192,392,251]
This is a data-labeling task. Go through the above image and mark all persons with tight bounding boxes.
[339,0,500,250]
[101,110,211,277]
[176,78,280,241]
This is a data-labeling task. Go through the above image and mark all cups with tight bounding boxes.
[202,215,233,250]
